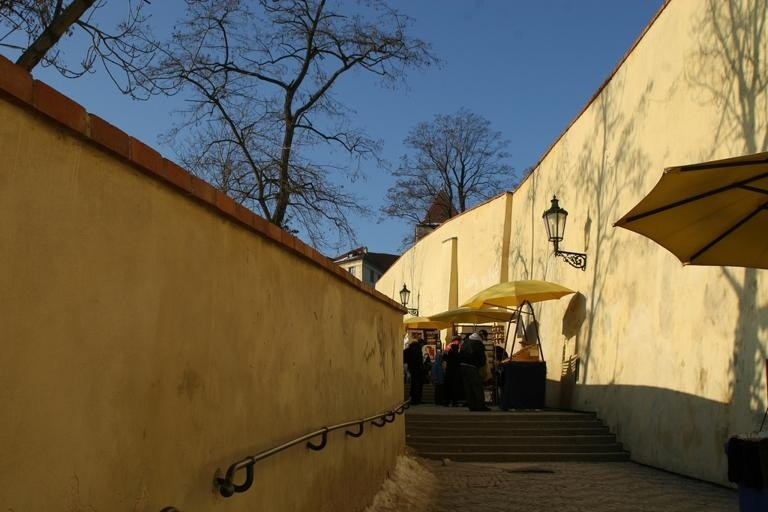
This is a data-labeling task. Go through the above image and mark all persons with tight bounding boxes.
[457,329,494,412]
[405,339,429,404]
[430,351,443,404]
[441,334,463,407]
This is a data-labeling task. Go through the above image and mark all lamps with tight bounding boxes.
[400,283,418,316]
[542,195,587,271]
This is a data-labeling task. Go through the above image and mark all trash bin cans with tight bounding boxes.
[725,440,768,512]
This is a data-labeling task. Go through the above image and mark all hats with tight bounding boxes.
[469,333,482,341]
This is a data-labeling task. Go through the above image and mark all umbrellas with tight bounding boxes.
[426,307,522,332]
[403,315,450,329]
[613,151,767,270]
[458,279,577,309]
